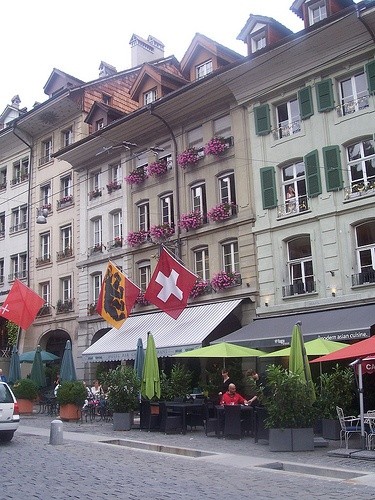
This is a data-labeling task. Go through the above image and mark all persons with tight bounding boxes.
[0,369,7,384]
[215,368,248,406]
[89,379,108,415]
[243,369,267,406]
[286,185,296,213]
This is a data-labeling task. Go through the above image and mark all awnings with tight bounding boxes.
[82,299,242,363]
[209,304,375,351]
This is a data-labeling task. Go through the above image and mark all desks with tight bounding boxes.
[358,413,375,419]
[165,402,203,435]
[216,405,254,439]
[150,400,184,407]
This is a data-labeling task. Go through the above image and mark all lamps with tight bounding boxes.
[37,209,47,224]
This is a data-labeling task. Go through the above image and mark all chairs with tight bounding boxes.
[80,394,116,424]
[335,405,375,451]
[139,392,267,443]
[40,393,60,415]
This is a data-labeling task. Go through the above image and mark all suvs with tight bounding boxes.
[0,381,21,442]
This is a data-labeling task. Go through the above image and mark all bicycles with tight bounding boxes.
[35,394,114,424]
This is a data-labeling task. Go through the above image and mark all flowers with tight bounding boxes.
[135,292,152,306]
[149,159,168,174]
[126,222,174,248]
[189,271,238,297]
[179,211,205,232]
[106,182,122,191]
[177,148,204,168]
[39,202,52,210]
[88,187,103,199]
[55,195,72,207]
[207,202,237,221]
[127,167,146,184]
[201,137,228,155]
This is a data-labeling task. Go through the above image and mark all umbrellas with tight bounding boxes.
[134,338,145,382]
[170,341,266,368]
[7,345,20,385]
[141,332,160,401]
[60,340,77,384]
[308,335,375,363]
[18,350,62,363]
[30,343,47,389]
[255,334,353,400]
[289,321,317,406]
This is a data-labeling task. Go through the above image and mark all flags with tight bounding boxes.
[0,279,46,331]
[94,260,141,329]
[145,247,198,320]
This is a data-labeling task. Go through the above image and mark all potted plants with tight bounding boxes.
[248,363,317,452]
[99,364,142,430]
[56,380,87,421]
[12,379,39,413]
[312,364,356,441]
